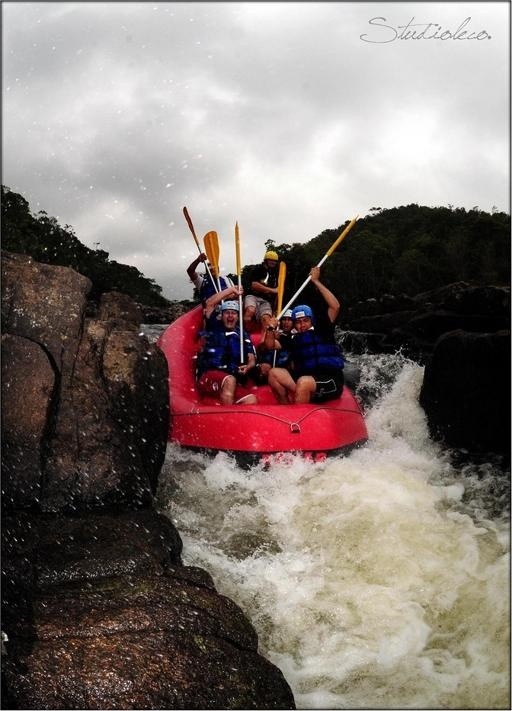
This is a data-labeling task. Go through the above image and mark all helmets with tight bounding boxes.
[264,251,278,261]
[222,301,239,311]
[292,305,313,319]
[282,308,293,318]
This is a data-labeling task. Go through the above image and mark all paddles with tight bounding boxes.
[268,214,359,329]
[273,261,288,367]
[233,222,244,365]
[183,206,226,305]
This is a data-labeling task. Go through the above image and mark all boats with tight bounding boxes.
[153,299,367,470]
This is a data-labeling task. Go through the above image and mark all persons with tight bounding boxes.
[198,283,274,407]
[238,251,297,378]
[268,267,345,406]
[186,253,237,301]
